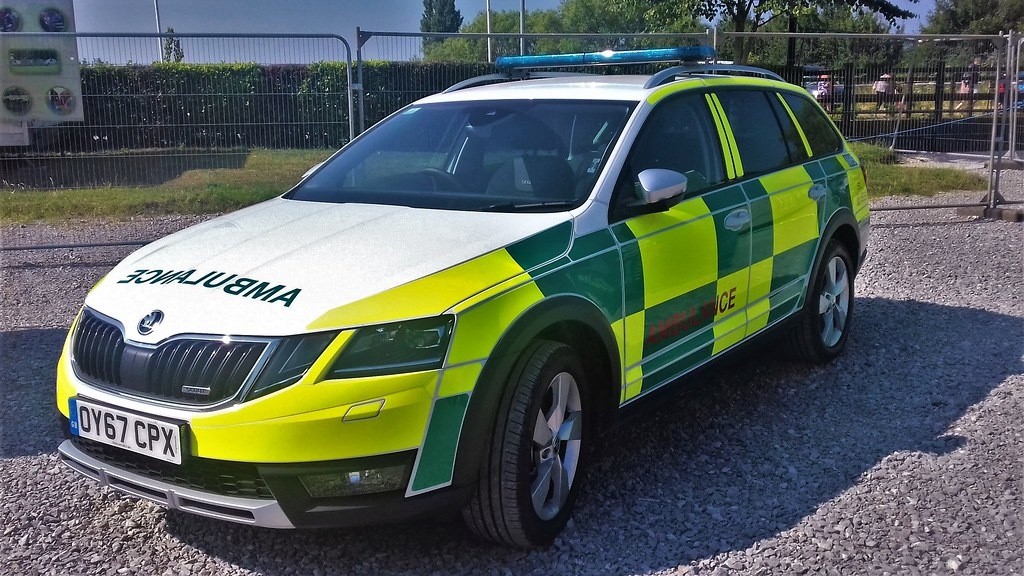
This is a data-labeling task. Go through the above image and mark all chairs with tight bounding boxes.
[486,120,576,199]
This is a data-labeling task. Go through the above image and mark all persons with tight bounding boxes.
[817,78,831,110]
[955,79,969,109]
[874,73,897,108]
[998,74,1007,108]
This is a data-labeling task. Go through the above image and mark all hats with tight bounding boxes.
[880,73,891,79]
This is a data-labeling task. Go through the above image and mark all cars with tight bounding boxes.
[993,70,1024,103]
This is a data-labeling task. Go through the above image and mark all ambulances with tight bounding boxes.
[49,50,873,554]
